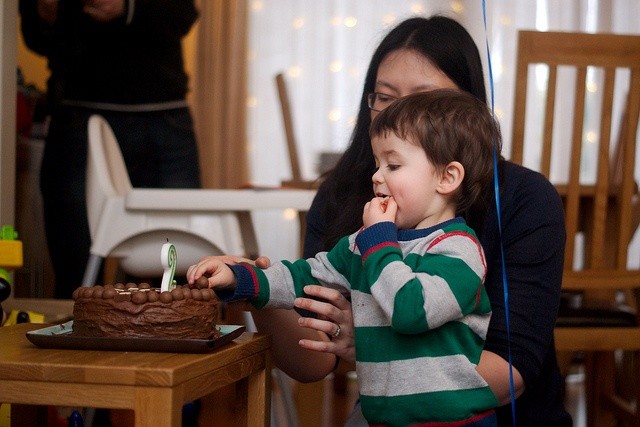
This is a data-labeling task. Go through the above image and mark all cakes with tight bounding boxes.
[73,238,222,339]
[381,196,391,213]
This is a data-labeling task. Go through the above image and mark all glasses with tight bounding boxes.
[368,92,397,112]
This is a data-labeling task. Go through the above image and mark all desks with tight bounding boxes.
[0,299,274,427]
[552,181,639,427]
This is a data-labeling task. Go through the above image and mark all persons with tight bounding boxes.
[16,1,204,301]
[184,87,502,426]
[197,14,574,427]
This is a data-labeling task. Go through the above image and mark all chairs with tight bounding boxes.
[512,29,639,426]
[81,114,301,427]
[275,71,329,258]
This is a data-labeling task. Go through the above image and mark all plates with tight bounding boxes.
[25,320,246,354]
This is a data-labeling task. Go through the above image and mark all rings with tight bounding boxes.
[332,323,341,338]
